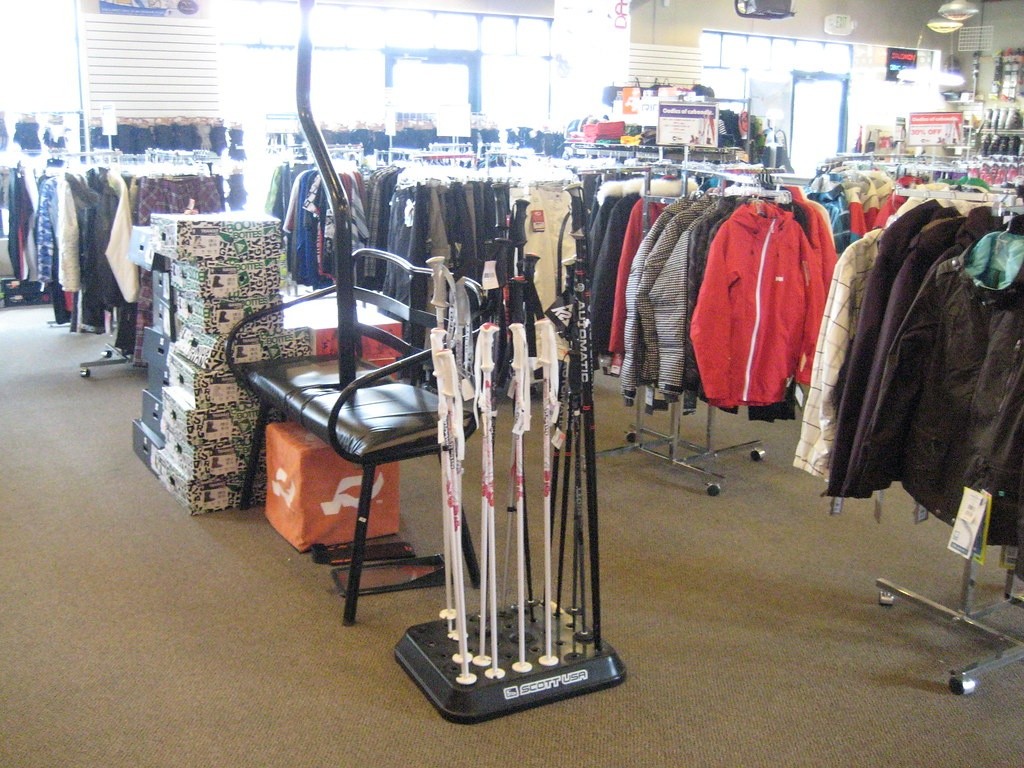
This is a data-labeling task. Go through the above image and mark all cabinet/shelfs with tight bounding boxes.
[972,128,1024,163]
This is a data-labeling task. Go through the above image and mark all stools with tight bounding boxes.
[265,422,399,551]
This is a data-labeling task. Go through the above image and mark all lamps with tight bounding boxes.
[734,0,796,20]
[937,0,980,20]
[926,16,964,34]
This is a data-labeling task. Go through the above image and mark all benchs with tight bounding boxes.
[226,249,480,627]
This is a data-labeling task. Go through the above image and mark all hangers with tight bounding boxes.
[623,157,1024,272]
[16,112,618,191]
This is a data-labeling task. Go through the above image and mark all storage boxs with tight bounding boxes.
[944,92,974,101]
[128,215,312,516]
